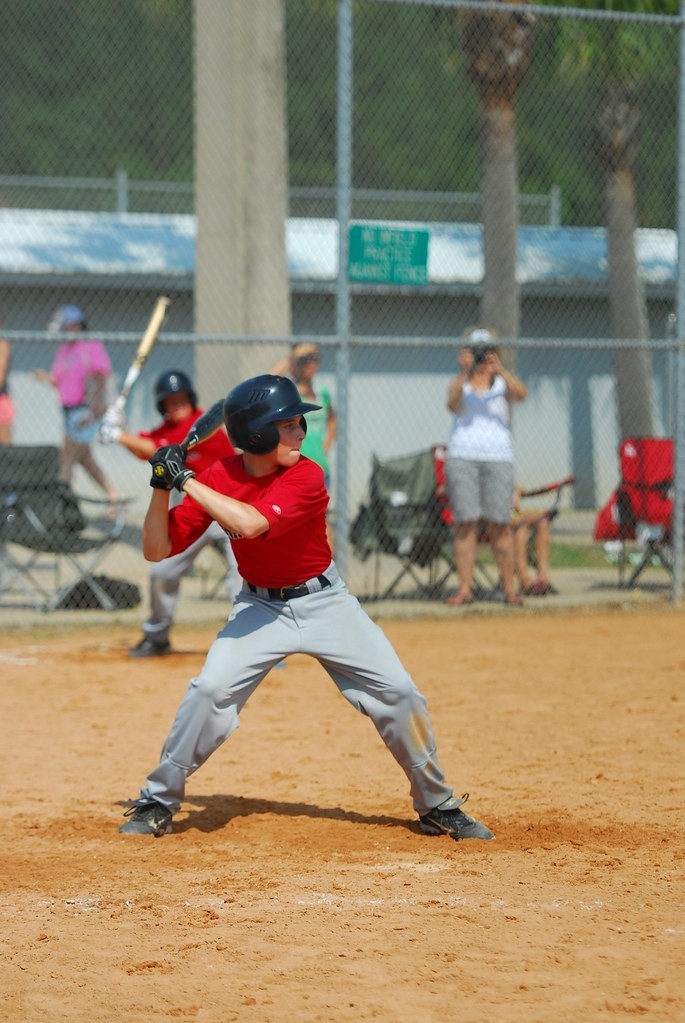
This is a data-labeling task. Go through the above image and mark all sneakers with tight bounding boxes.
[118,800,174,834]
[419,792,495,841]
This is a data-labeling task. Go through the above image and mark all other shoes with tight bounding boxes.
[447,589,476,605]
[130,636,172,659]
[523,580,560,596]
[505,593,524,606]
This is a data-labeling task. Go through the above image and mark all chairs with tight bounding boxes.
[351,452,456,598]
[595,440,674,586]
[0,443,135,610]
[435,447,578,598]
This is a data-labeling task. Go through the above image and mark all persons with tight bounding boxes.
[32,303,120,522]
[119,376,496,841]
[100,370,246,659]
[509,485,561,595]
[445,324,526,606]
[0,341,16,447]
[270,341,336,562]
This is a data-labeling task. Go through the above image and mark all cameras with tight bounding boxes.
[468,345,493,364]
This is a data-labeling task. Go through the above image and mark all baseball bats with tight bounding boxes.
[152,397,225,478]
[103,295,171,441]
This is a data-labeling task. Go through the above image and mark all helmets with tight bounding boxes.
[223,375,323,455]
[155,369,197,418]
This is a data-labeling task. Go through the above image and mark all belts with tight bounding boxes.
[246,574,331,603]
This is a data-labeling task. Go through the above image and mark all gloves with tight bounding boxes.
[148,442,195,492]
[98,406,127,444]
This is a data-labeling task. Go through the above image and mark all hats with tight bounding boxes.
[463,329,496,350]
[53,303,85,328]
